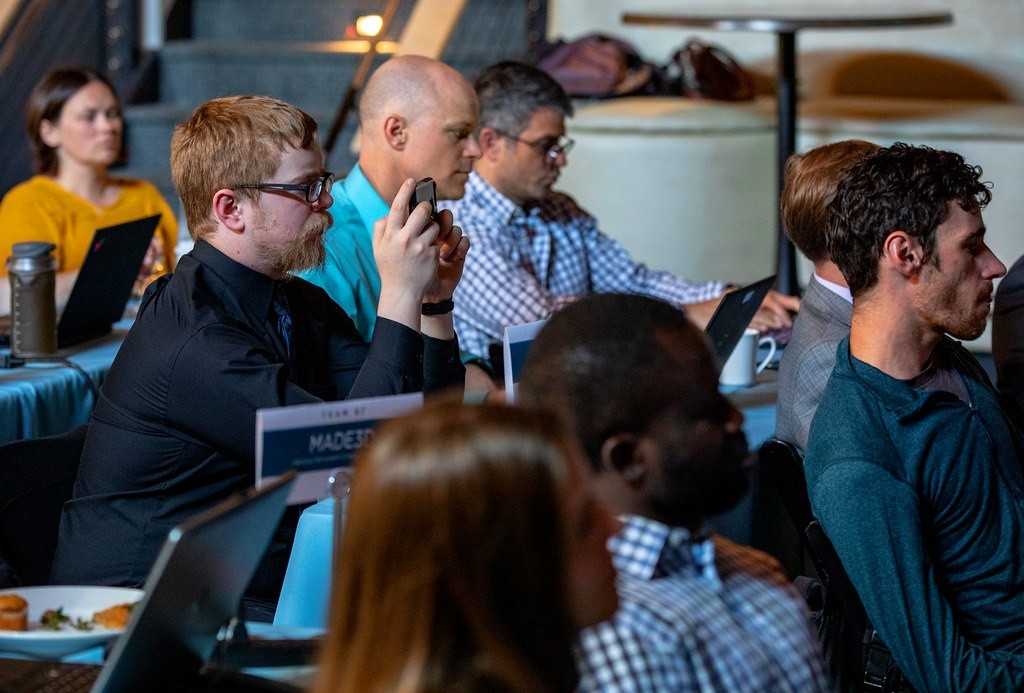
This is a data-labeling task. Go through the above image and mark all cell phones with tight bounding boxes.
[409,178,439,225]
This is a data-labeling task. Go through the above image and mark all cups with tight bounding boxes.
[718,327,776,386]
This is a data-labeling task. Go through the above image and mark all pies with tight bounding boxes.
[92,603,133,628]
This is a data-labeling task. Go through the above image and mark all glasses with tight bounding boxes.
[494,129,574,166]
[235,172,335,203]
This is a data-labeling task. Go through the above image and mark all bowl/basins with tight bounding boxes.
[0,586,146,660]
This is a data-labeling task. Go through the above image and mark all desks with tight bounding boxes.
[0,287,785,693]
[618,8,953,299]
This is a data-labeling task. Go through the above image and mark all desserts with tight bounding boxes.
[0,594,28,631]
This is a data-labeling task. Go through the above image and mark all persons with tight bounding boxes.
[0,64,178,316]
[993,255,1024,408]
[801,142,1024,693]
[774,140,884,456]
[314,400,624,693]
[435,59,802,361]
[286,55,505,399]
[518,291,835,693]
[52,95,469,625]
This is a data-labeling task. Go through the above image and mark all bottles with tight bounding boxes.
[7,242,58,359]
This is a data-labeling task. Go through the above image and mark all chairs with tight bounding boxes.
[1,425,88,588]
[759,438,868,693]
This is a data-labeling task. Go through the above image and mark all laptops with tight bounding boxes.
[702,273,778,375]
[0,213,163,352]
[1,469,300,693]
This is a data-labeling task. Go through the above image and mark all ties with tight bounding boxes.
[271,292,295,369]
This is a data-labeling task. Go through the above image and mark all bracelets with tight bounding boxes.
[420,297,453,315]
